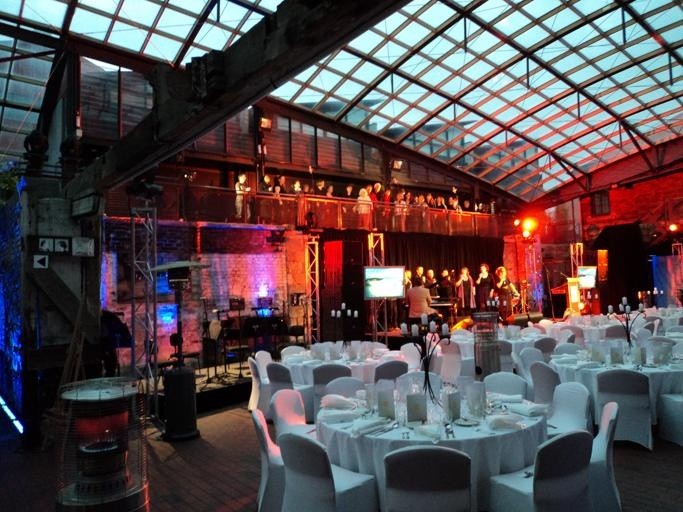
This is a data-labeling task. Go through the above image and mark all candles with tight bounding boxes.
[486,296,507,306]
[608,296,645,314]
[647,287,664,295]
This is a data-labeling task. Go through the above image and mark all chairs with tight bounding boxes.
[250,407,283,510]
[383,445,472,512]
[592,401,622,511]
[277,431,379,512]
[488,430,592,512]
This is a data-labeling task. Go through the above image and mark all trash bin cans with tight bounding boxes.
[474,344,499,381]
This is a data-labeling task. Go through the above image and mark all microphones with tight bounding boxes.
[269,306,279,310]
[222,308,231,313]
[212,309,222,314]
[250,307,262,310]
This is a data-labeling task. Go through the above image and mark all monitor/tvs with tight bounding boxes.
[363,266,405,300]
[577,266,597,289]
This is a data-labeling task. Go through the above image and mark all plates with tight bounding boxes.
[454,419,478,426]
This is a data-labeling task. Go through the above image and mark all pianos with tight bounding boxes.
[396,296,454,330]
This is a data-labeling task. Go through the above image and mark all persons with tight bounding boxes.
[39,240,51,252]
[56,240,68,252]
[387,264,522,331]
[234,172,498,225]
